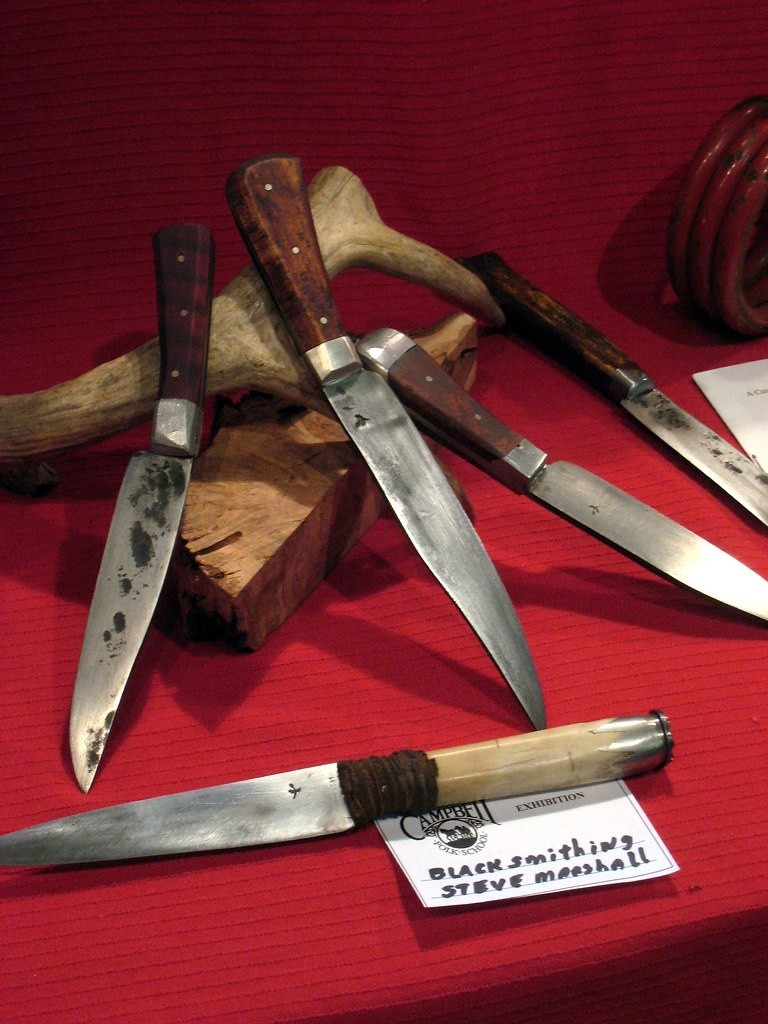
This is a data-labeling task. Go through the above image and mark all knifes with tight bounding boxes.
[459,251,768,532]
[65,221,217,794]
[0,711,677,868]
[353,319,768,623]
[224,153,547,730]
